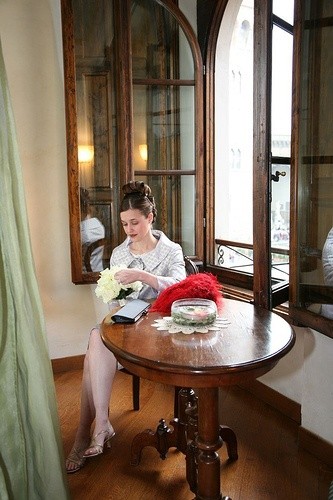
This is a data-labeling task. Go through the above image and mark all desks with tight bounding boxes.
[99,298,296,500]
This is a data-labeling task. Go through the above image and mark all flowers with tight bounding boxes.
[94,264,144,301]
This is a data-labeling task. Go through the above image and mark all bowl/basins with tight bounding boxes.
[171,298,217,328]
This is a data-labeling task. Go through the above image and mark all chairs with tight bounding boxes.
[84,238,109,272]
[135,256,200,411]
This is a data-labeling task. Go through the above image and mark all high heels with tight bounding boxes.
[82,424,116,458]
[65,446,89,474]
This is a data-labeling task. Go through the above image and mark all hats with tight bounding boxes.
[150,297,231,335]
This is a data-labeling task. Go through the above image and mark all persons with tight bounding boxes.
[80,188,105,274]
[322,226,333,324]
[64,180,186,474]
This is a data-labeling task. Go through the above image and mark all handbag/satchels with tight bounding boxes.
[110,299,152,323]
